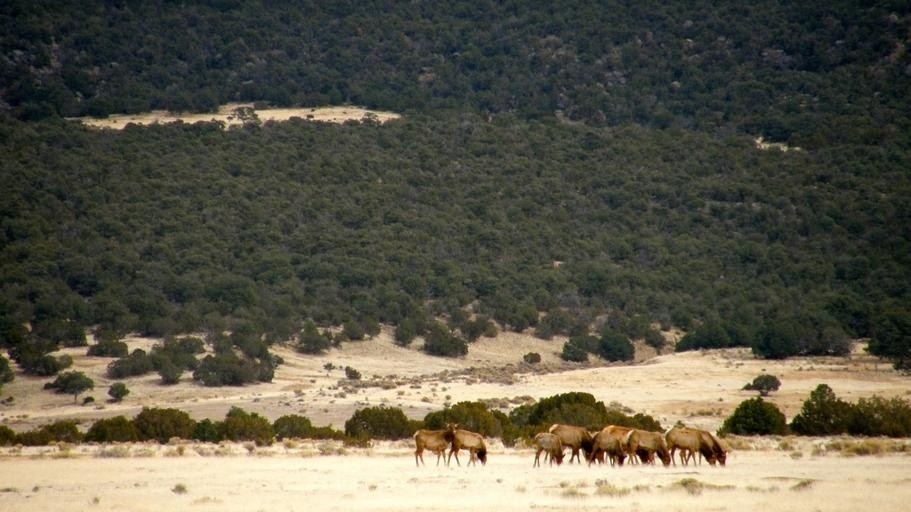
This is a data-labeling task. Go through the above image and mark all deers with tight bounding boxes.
[414,422,487,466]
[533,423,727,468]
